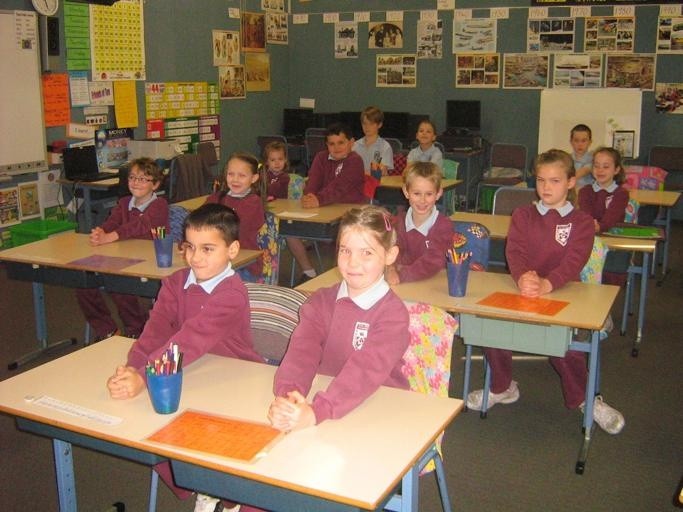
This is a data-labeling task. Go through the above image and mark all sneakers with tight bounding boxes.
[578,392,625,436]
[464,377,521,412]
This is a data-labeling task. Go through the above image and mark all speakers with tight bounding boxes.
[39,15,61,72]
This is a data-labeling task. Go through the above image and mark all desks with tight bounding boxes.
[291,266,623,476]
[302,170,464,214]
[0,335,466,512]
[449,149,484,211]
[447,213,656,359]
[54,167,120,230]
[1,231,261,373]
[172,193,364,288]
[506,179,682,278]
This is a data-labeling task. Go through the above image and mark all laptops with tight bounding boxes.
[63,145,119,182]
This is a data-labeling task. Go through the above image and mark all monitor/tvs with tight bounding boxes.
[446,99,480,135]
[283,108,429,144]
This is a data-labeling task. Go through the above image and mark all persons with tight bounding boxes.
[201,150,265,285]
[105,201,261,402]
[405,119,444,172]
[74,156,170,340]
[576,146,629,236]
[565,123,596,189]
[255,137,292,201]
[282,119,367,288]
[350,105,396,181]
[462,146,625,435]
[235,204,412,512]
[384,161,456,287]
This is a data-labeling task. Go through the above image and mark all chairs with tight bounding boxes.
[471,142,529,213]
[621,200,641,226]
[621,165,670,193]
[440,159,460,211]
[647,146,682,192]
[362,171,379,204]
[490,187,541,217]
[442,220,492,272]
[164,204,190,244]
[242,283,309,365]
[287,173,305,200]
[167,124,445,163]
[387,154,408,176]
[400,302,459,510]
[254,210,281,287]
[576,237,616,342]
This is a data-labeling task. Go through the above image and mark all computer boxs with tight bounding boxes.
[439,134,481,151]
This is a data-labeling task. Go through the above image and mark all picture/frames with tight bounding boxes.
[584,15,635,53]
[375,54,418,88]
[502,53,550,90]
[455,53,500,89]
[552,53,603,88]
[526,16,576,53]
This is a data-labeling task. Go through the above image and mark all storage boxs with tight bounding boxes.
[126,136,177,163]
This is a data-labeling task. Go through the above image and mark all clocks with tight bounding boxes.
[32,0,59,17]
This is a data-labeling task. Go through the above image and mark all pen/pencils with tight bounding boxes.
[145,342,183,413]
[151,226,172,267]
[445,247,472,298]
[371,158,382,171]
